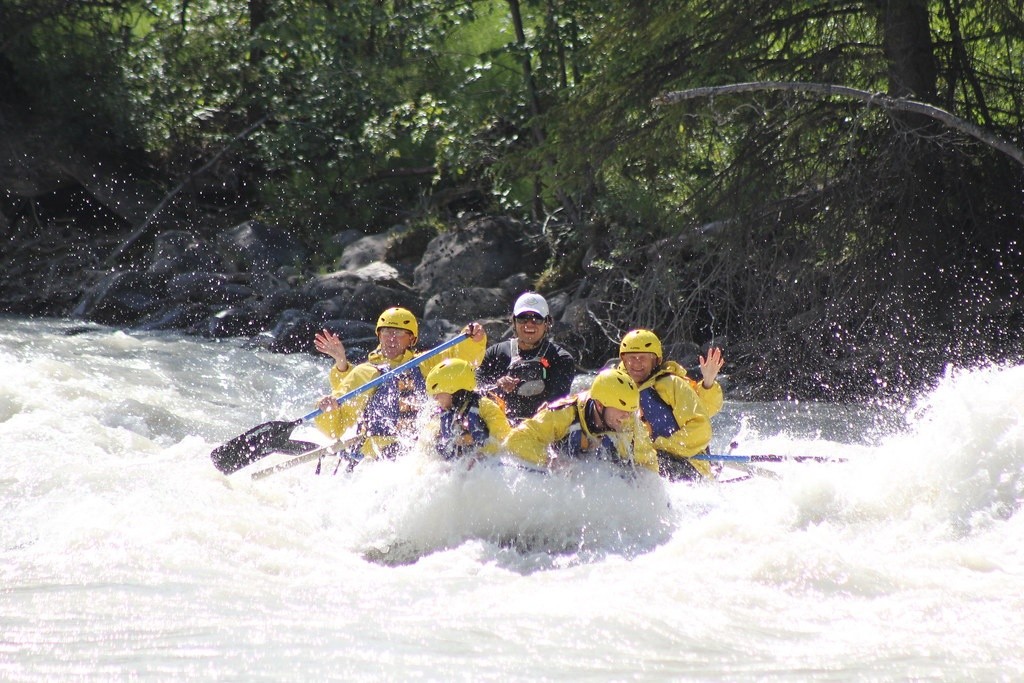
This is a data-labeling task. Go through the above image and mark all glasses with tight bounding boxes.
[379,328,412,336]
[515,313,544,325]
[622,353,657,361]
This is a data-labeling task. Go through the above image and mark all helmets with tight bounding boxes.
[375,308,418,347]
[590,369,640,412]
[619,328,662,364]
[514,292,550,318]
[426,359,476,394]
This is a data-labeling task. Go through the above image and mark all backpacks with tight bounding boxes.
[505,337,551,418]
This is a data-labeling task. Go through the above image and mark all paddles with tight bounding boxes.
[207,323,479,484]
[693,452,848,465]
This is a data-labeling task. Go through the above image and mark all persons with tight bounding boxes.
[508,369,659,477]
[313,308,487,467]
[418,358,510,471]
[476,292,575,427]
[617,329,724,482]
